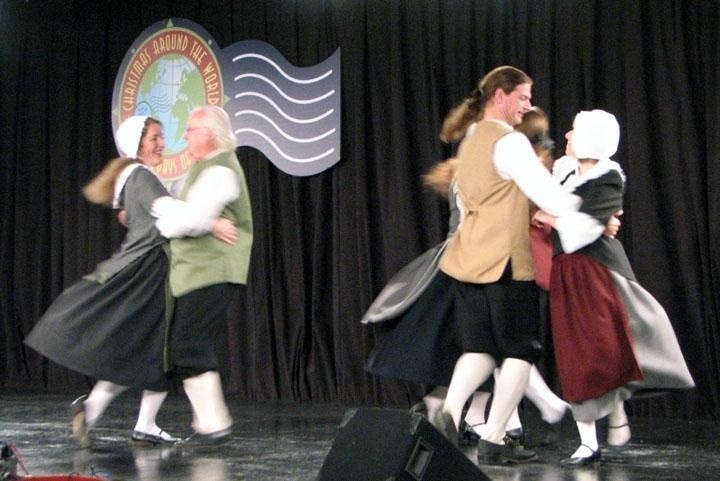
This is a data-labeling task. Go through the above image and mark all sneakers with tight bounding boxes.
[67,394,93,449]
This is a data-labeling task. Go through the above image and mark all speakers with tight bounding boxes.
[317,407,492,481]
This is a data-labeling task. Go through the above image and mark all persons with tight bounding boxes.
[112,105,256,454]
[21,116,242,451]
[358,66,568,462]
[531,109,695,465]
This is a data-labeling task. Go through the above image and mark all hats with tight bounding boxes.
[114,115,148,160]
[571,108,622,161]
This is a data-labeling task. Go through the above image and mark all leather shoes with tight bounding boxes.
[174,424,236,449]
[559,441,603,468]
[409,400,564,466]
[131,428,182,446]
[600,423,634,455]
